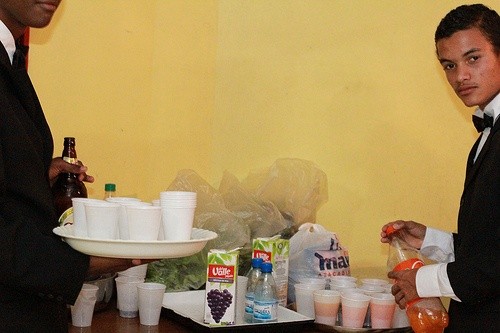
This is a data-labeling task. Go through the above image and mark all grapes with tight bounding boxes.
[207,289,233,323]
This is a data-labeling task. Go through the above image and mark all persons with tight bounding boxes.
[381,4,500,333]
[0,0,161,332]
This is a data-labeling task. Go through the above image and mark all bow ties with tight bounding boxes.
[12,39,29,75]
[472,113,493,132]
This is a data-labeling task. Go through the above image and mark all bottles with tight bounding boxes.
[244,258,263,322]
[386,226,449,333]
[51,137,87,224]
[104,184,116,200]
[252,263,278,323]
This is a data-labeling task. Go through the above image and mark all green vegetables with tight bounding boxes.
[144,246,210,293]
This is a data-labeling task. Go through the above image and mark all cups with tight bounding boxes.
[107,198,140,238]
[152,200,161,206]
[72,198,106,237]
[381,284,411,328]
[294,284,320,319]
[313,290,341,326]
[126,206,161,241]
[117,272,145,308]
[137,284,166,325]
[83,202,119,238]
[360,277,390,295]
[122,202,152,238]
[341,294,369,327]
[330,276,356,292]
[369,293,396,328]
[160,191,197,241]
[71,284,99,327]
[300,278,326,289]
[115,277,144,318]
